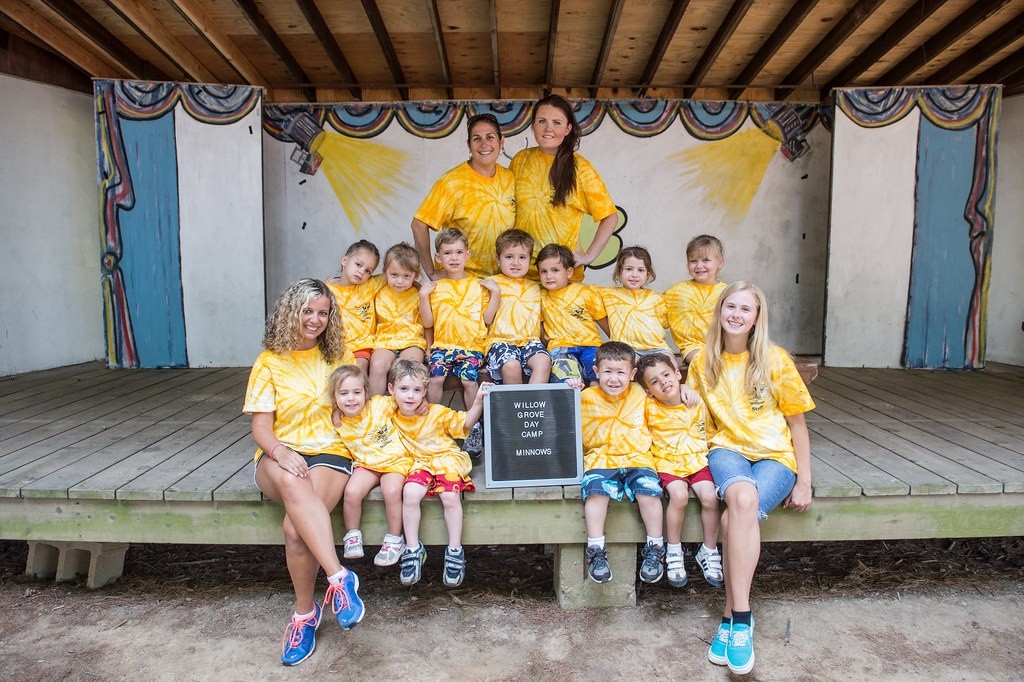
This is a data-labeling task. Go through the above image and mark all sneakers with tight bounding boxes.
[442,546,466,587]
[322,565,365,631]
[462,422,483,458]
[665,551,687,587]
[707,622,729,665]
[280,600,323,666]
[398,541,427,586]
[725,613,755,674]
[585,545,612,583]
[342,529,364,559]
[373,534,406,567]
[639,540,666,584]
[695,544,724,587]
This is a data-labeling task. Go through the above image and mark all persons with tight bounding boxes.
[482,227,551,384]
[564,340,700,584]
[685,280,817,674]
[418,227,501,459]
[635,353,724,588]
[328,365,431,566]
[243,276,366,665]
[411,112,516,280]
[507,93,618,284]
[331,357,495,587]
[661,234,818,385]
[324,238,422,388]
[533,244,679,386]
[367,239,434,400]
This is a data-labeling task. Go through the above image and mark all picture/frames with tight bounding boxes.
[481,383,584,490]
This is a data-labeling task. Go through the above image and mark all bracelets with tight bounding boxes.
[270,443,285,460]
